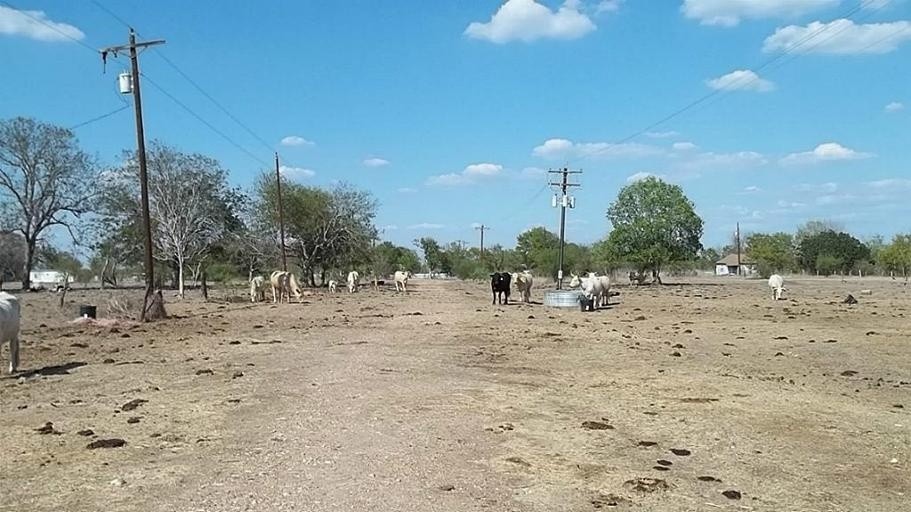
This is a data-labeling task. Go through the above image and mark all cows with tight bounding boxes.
[768,274,787,300]
[0,291,20,375]
[569,270,610,311]
[270,270,307,303]
[627,271,659,288]
[394,271,410,292]
[251,276,266,302]
[489,272,512,305]
[347,271,360,294]
[507,271,533,303]
[328,280,339,294]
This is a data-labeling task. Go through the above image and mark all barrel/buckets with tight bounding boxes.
[80,305,97,319]
